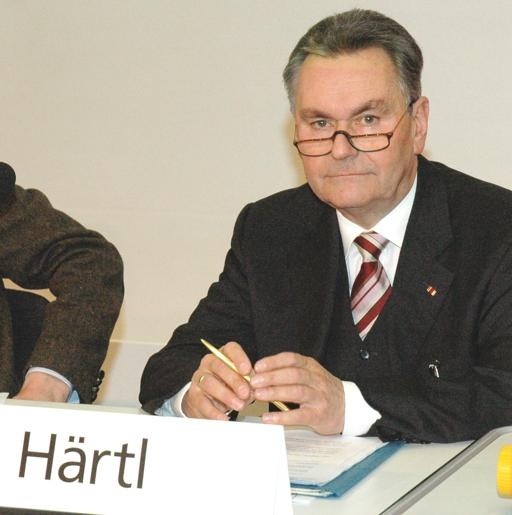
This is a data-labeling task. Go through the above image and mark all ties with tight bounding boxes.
[350,232,393,342]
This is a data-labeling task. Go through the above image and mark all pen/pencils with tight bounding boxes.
[200,336,290,412]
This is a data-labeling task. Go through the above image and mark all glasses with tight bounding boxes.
[293,98,419,157]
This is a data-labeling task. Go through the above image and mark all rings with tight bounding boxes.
[199,373,210,384]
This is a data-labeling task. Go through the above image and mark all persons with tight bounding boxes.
[139,9,512,445]
[0,161,124,404]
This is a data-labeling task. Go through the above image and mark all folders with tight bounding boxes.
[290,438,405,500]
